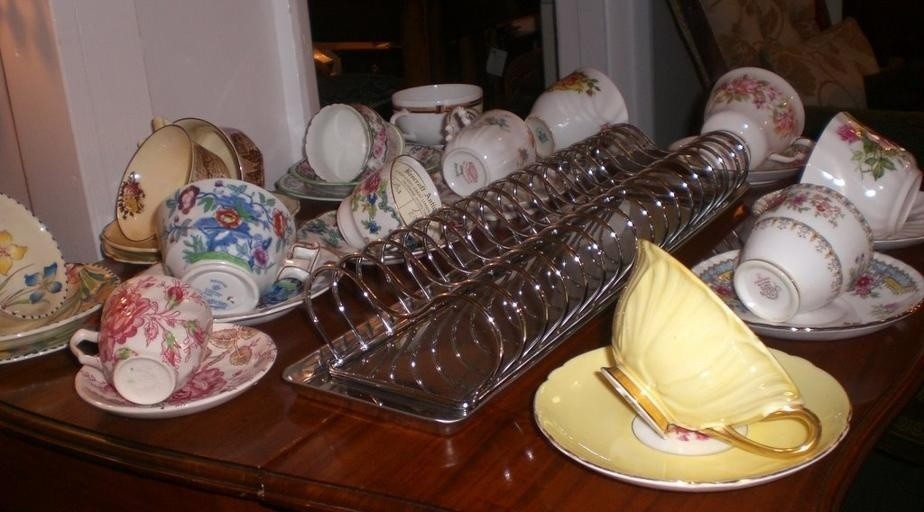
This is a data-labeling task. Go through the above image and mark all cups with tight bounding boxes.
[600,238,823,459]
[151,178,321,318]
[272,67,631,266]
[731,187,874,323]
[798,110,919,242]
[70,274,213,407]
[701,66,808,173]
[114,114,262,243]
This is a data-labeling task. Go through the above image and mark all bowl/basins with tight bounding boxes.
[0,191,70,322]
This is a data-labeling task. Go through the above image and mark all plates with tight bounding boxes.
[297,208,476,268]
[74,322,276,419]
[101,191,301,268]
[749,185,924,245]
[531,341,855,491]
[689,247,923,337]
[2,261,124,368]
[669,135,819,185]
[137,242,345,330]
